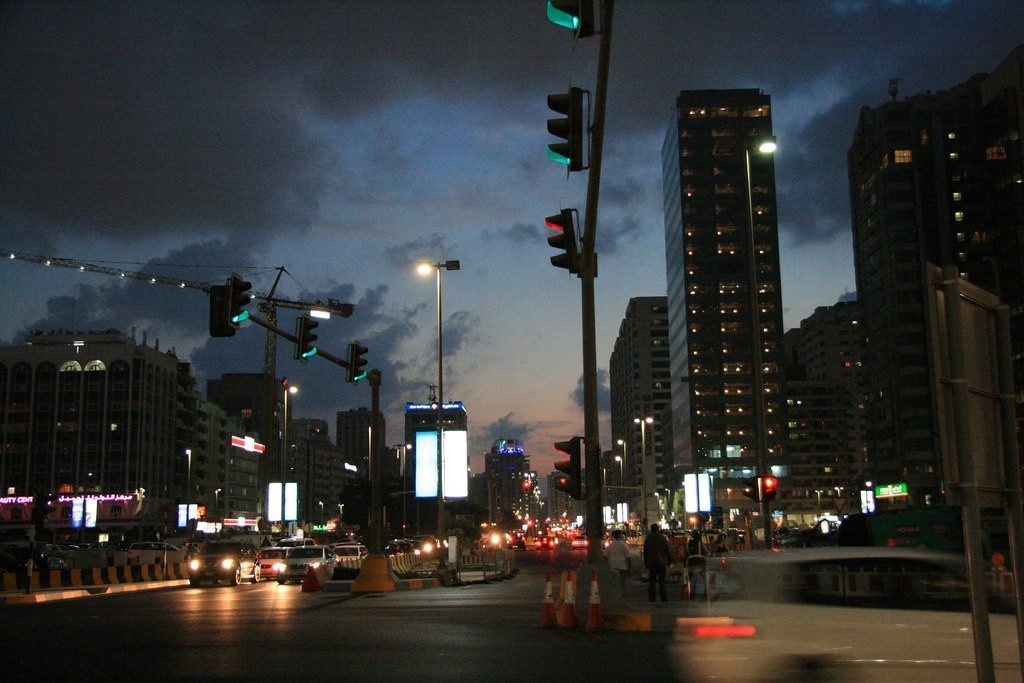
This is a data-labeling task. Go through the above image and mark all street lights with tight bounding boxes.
[633,417,655,529]
[743,139,779,561]
[279,386,301,535]
[418,258,462,567]
[617,439,627,530]
[834,487,845,500]
[213,488,222,535]
[185,449,191,538]
[814,489,824,522]
[318,501,324,533]
[615,455,625,533]
[664,488,671,523]
[600,465,607,535]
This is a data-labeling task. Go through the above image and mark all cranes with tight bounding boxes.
[0,243,356,519]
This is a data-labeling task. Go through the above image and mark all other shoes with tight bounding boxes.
[648,597,656,601]
[661,597,667,600]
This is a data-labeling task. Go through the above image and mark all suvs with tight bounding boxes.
[187,538,263,586]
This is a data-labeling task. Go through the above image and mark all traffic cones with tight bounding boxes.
[583,569,609,631]
[555,570,583,634]
[532,571,559,629]
[678,562,694,601]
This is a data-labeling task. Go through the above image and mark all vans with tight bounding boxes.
[662,545,1023,683]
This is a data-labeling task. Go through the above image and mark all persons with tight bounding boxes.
[607,530,632,589]
[712,534,728,553]
[644,524,672,602]
[685,529,707,600]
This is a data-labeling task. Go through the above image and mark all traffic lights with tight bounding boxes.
[689,517,696,524]
[346,342,369,386]
[293,316,318,364]
[741,476,761,505]
[763,478,778,499]
[545,78,585,181]
[225,273,252,331]
[554,437,582,501]
[545,0,595,50]
[544,205,578,275]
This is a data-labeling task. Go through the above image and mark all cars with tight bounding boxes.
[483,523,558,550]
[572,535,587,550]
[258,547,293,582]
[0,525,448,580]
[275,544,337,585]
